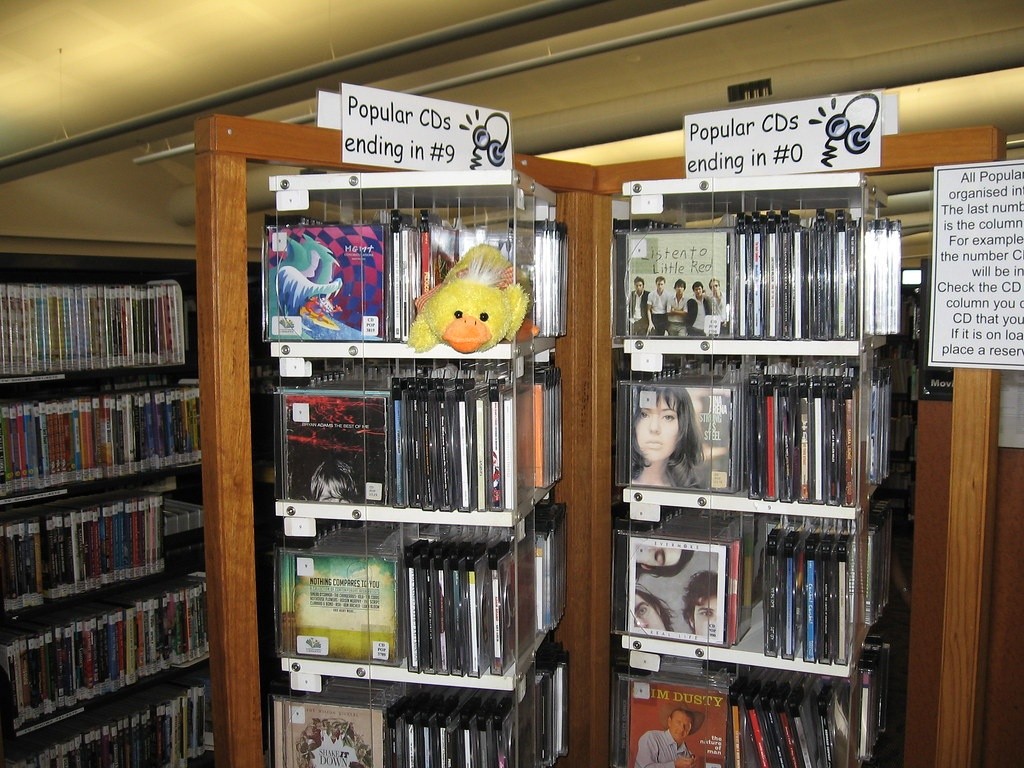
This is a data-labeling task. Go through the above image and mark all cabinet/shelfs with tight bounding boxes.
[190,110,600,768]
[589,123,1008,768]
[0,250,286,768]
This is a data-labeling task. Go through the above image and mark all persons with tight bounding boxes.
[635,544,695,583]
[310,459,361,506]
[890,544,912,607]
[633,708,699,768]
[630,384,706,487]
[628,275,726,336]
[634,582,674,632]
[681,570,719,638]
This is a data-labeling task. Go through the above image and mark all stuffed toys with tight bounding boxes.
[406,244,540,354]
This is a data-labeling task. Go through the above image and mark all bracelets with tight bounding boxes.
[898,585,911,594]
